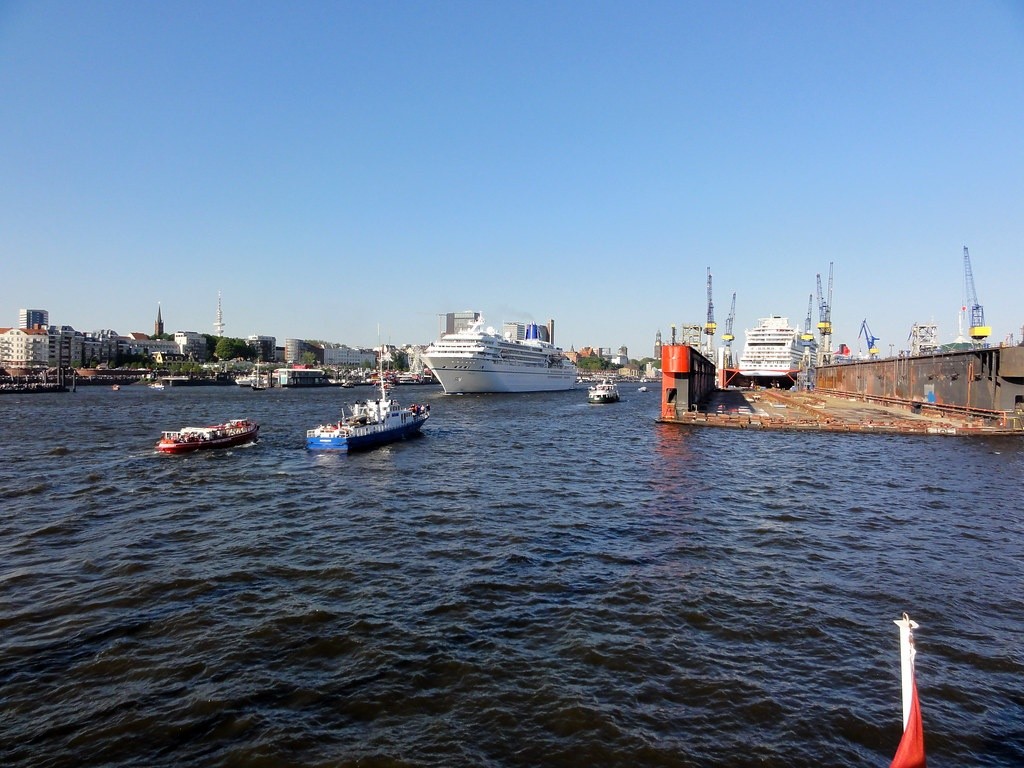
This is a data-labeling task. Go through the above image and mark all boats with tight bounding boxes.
[341,380,355,388]
[399,378,420,385]
[305,321,430,454]
[587,379,620,403]
[154,416,260,455]
[234,357,269,390]
[113,384,121,391]
[147,382,165,391]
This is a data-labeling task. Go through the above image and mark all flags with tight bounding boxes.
[890,667,926,768]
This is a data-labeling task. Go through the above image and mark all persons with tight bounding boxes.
[409,403,430,417]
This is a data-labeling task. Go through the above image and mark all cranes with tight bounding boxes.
[801,261,834,368]
[704,267,717,362]
[962,244,991,350]
[722,290,737,370]
[856,319,880,361]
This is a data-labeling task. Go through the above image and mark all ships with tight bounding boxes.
[739,314,804,377]
[419,311,578,395]
